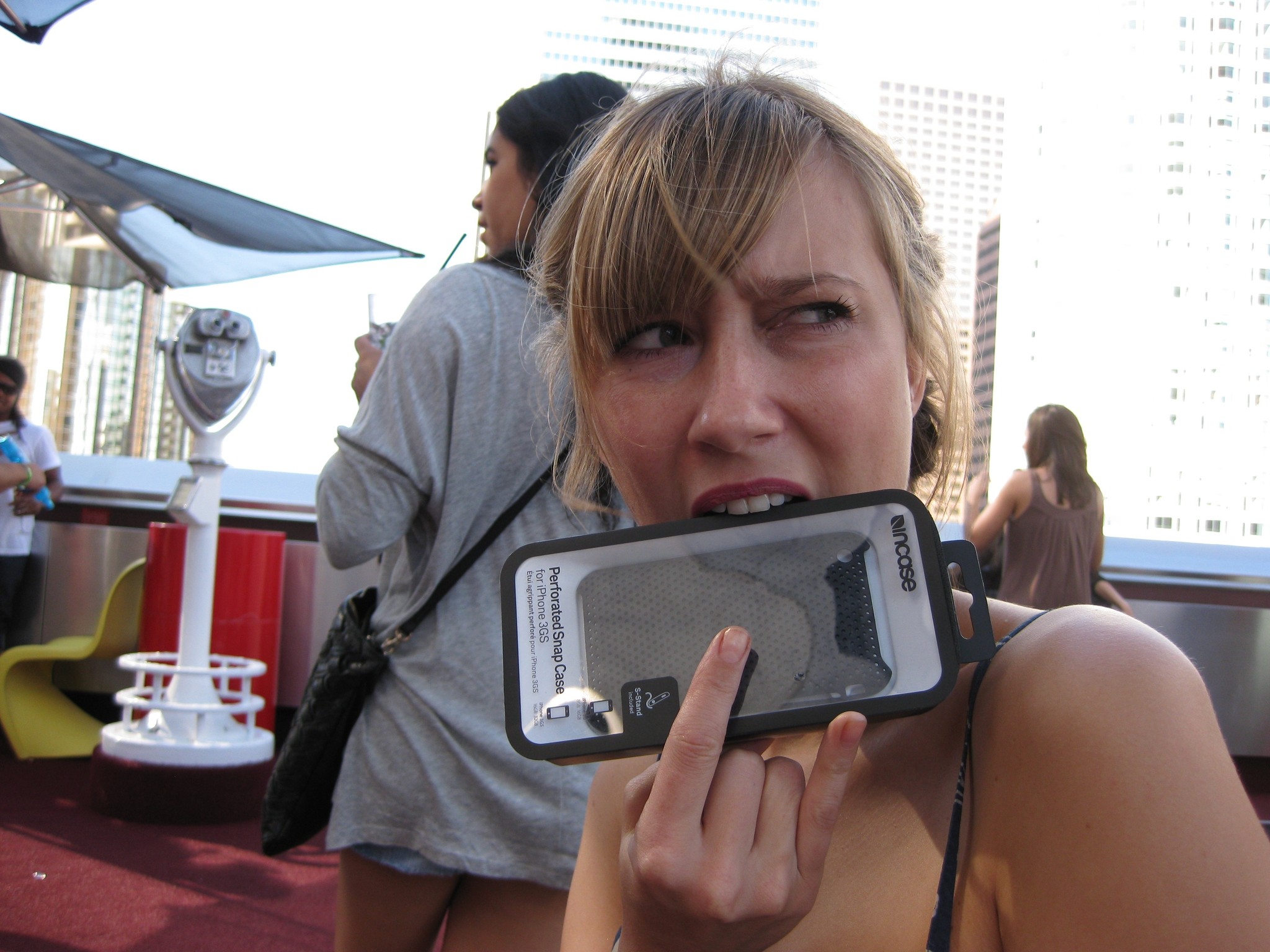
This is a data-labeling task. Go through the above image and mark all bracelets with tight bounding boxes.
[16,463,32,490]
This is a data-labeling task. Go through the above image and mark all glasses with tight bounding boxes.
[0,381,21,396]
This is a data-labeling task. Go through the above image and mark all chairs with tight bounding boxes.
[0,555,148,760]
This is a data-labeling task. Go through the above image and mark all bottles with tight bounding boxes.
[0,434,54,511]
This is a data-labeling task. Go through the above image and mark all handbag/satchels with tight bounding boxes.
[258,585,406,857]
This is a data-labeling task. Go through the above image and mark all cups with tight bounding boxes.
[369,289,418,353]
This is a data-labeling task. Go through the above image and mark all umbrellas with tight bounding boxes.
[0,0,94,45]
[0,113,425,294]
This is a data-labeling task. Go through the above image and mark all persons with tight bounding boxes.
[966,521,1133,612]
[966,406,1101,606]
[316,73,636,952]
[0,357,63,652]
[517,53,1270,952]
